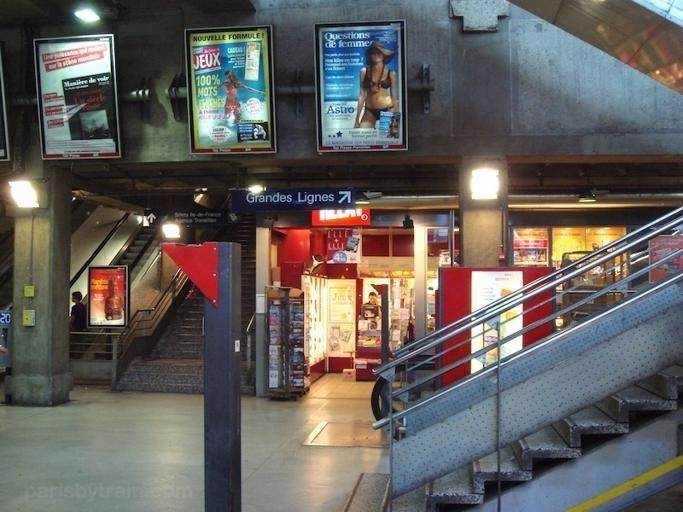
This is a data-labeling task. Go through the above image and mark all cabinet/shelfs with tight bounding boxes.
[266,288,305,402]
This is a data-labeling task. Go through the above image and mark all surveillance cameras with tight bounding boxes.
[363,191,382,199]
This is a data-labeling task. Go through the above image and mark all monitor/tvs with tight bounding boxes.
[361,305,379,318]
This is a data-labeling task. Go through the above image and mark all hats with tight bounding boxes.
[365,42,394,65]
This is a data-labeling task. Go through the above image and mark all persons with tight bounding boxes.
[358,291,382,345]
[252,124,266,141]
[385,115,399,138]
[215,69,265,124]
[351,40,399,129]
[67,290,86,331]
